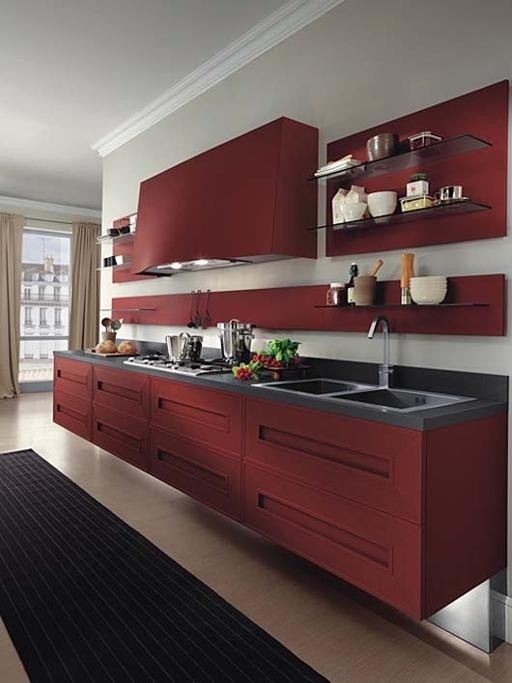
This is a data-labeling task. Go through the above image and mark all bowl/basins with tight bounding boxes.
[105,227,120,237]
[366,132,401,170]
[341,202,367,225]
[119,227,130,233]
[409,274,447,306]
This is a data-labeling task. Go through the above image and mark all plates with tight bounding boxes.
[434,197,469,206]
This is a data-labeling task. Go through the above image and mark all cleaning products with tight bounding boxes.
[401,252,415,304]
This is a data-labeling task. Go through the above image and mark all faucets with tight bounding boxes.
[367,314,395,388]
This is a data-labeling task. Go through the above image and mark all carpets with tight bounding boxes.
[0,448,332,683]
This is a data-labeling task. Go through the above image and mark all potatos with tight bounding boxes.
[95,339,117,353]
[117,340,136,353]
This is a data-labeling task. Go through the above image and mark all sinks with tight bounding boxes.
[251,377,375,408]
[330,386,477,415]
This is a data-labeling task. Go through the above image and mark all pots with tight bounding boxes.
[216,317,257,363]
[165,333,204,362]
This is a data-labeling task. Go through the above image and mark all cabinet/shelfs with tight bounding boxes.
[306,133,492,309]
[95,212,138,283]
[52,357,506,623]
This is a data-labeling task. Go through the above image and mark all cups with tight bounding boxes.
[435,185,462,198]
[353,276,376,307]
[102,331,116,343]
[327,282,345,305]
[103,254,124,267]
[367,190,398,224]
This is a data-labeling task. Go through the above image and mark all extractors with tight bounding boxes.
[131,115,320,277]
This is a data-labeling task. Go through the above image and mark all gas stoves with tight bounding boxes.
[124,350,231,377]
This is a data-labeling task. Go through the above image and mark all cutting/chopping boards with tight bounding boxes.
[83,349,141,358]
